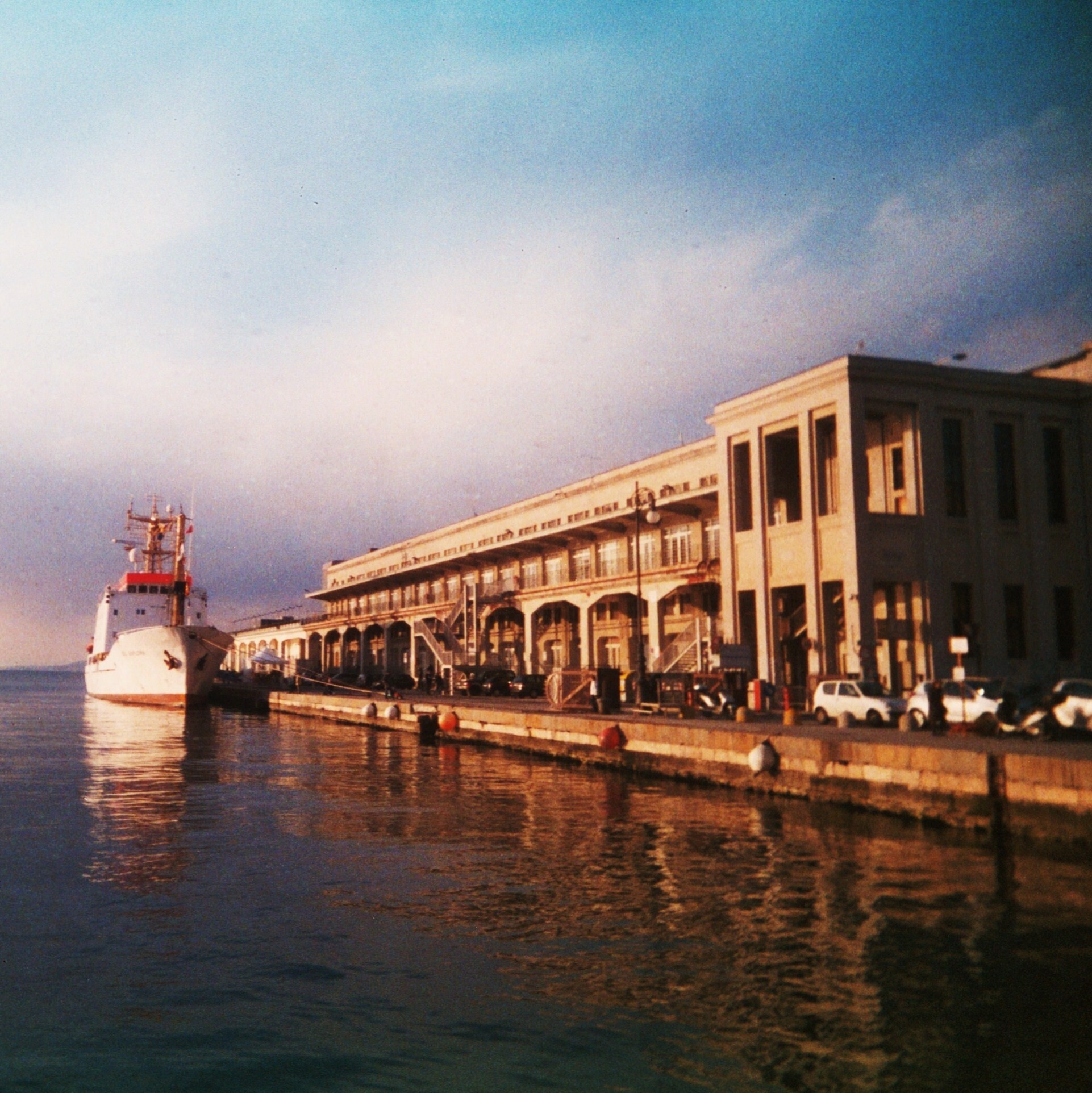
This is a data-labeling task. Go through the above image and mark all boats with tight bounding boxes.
[84,481,234,709]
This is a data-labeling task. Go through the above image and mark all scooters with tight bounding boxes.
[994,690,1068,743]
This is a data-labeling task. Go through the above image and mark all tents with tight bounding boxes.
[250,647,289,664]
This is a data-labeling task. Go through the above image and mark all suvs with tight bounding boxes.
[454,669,515,698]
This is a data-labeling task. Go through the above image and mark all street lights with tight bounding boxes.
[629,479,661,717]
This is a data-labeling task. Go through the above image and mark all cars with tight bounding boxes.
[906,675,1003,734]
[813,680,907,729]
[321,670,417,690]
[1049,678,1092,740]
[510,674,547,700]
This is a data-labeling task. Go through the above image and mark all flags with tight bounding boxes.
[186,525,193,534]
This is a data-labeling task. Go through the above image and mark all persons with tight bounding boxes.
[293,674,301,692]
[322,677,334,695]
[590,674,597,713]
[928,680,949,737]
[416,664,444,695]
[382,670,394,699]
